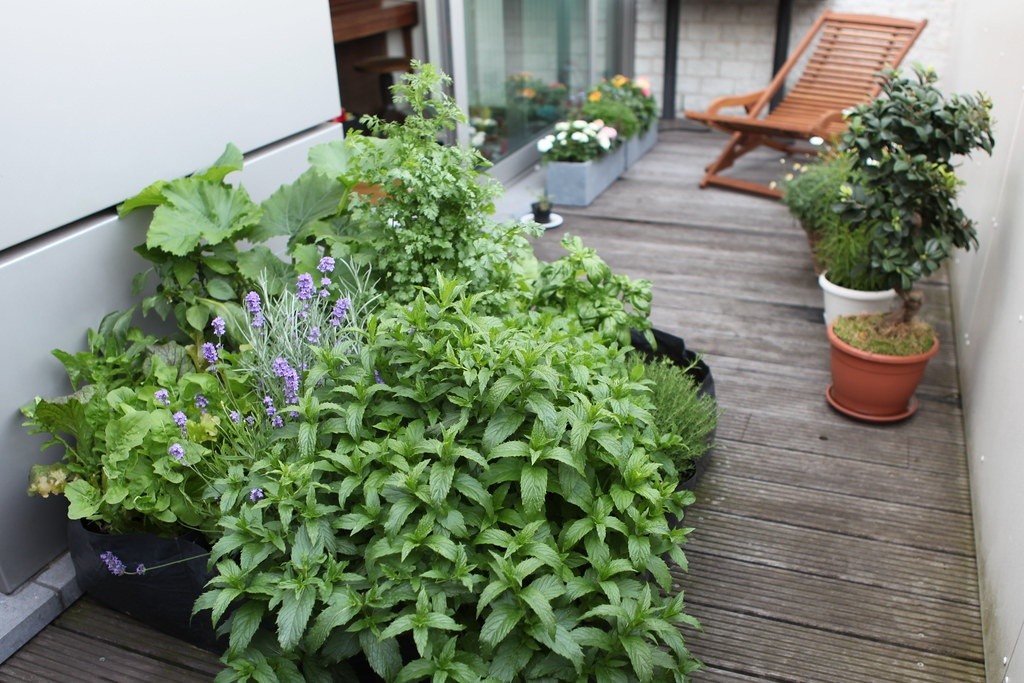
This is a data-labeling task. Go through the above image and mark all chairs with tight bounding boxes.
[685,9,929,204]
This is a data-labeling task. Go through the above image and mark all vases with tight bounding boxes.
[547,143,625,208]
[622,117,660,170]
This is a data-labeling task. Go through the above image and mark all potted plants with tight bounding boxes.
[13,304,258,657]
[825,314,942,424]
[778,61,996,330]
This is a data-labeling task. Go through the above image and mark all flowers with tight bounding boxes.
[509,71,567,121]
[97,251,390,577]
[580,75,662,144]
[536,117,623,164]
